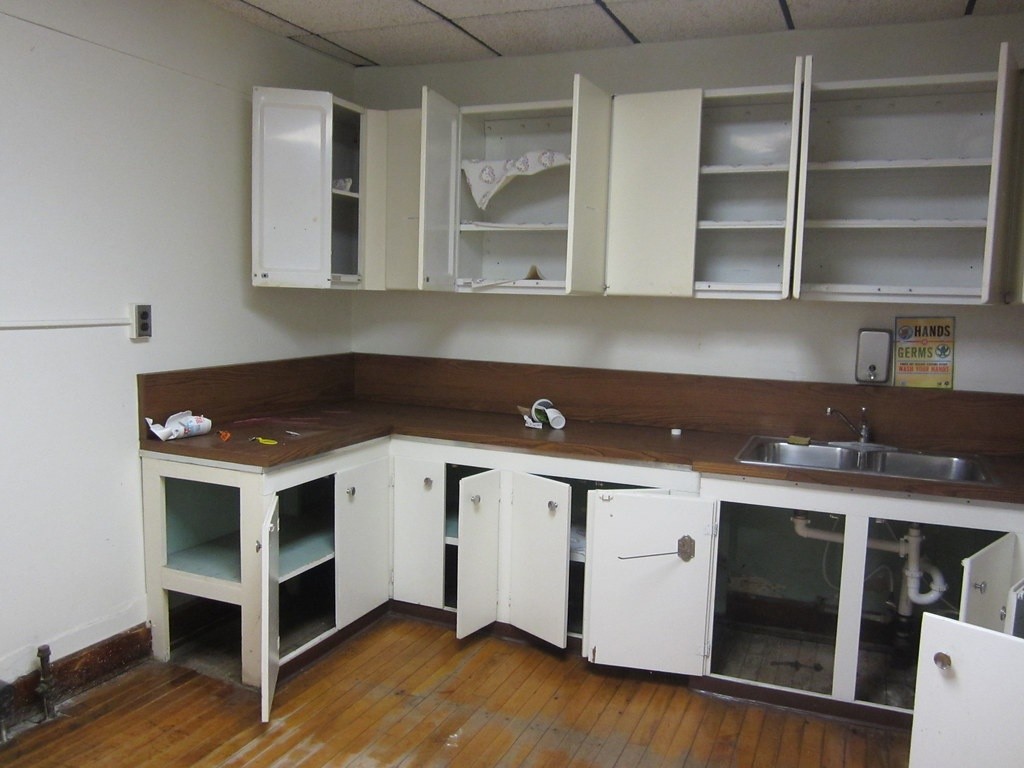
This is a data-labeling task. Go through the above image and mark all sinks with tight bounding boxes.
[859,443,1002,489]
[733,435,867,482]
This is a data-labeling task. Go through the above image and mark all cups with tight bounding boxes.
[532,398,566,428]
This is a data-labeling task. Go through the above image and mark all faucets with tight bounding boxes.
[825,404,869,444]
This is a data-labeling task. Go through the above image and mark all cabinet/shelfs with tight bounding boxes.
[237,39,1023,317]
[135,396,1023,766]
